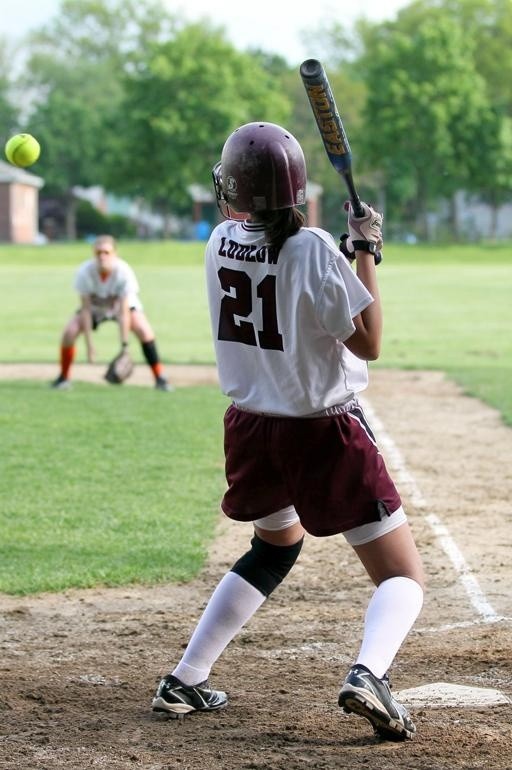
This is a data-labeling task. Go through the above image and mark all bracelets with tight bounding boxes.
[121,341,128,347]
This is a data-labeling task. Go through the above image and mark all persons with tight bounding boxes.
[49,234,174,392]
[149,122,431,741]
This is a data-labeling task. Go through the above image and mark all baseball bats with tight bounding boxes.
[300,59,382,264]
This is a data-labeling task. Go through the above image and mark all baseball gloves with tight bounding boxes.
[105,353,131,383]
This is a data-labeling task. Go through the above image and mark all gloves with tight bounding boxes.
[338,202,384,263]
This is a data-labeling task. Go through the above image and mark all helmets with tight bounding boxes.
[210,119,308,220]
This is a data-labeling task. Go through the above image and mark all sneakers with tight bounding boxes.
[336,663,417,744]
[149,672,231,720]
[154,376,176,392]
[50,376,71,391]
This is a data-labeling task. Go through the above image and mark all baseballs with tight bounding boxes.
[5,133,41,168]
